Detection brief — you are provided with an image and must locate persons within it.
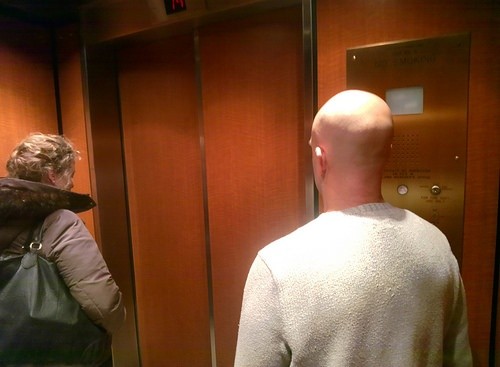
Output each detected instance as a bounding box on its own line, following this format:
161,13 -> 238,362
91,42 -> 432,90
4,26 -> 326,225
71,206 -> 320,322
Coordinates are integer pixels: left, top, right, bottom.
0,133 -> 125,367
234,90 -> 473,367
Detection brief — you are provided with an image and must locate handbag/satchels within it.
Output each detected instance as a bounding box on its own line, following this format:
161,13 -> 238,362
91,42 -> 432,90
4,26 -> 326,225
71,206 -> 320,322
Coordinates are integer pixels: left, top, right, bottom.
0,212 -> 113,367
